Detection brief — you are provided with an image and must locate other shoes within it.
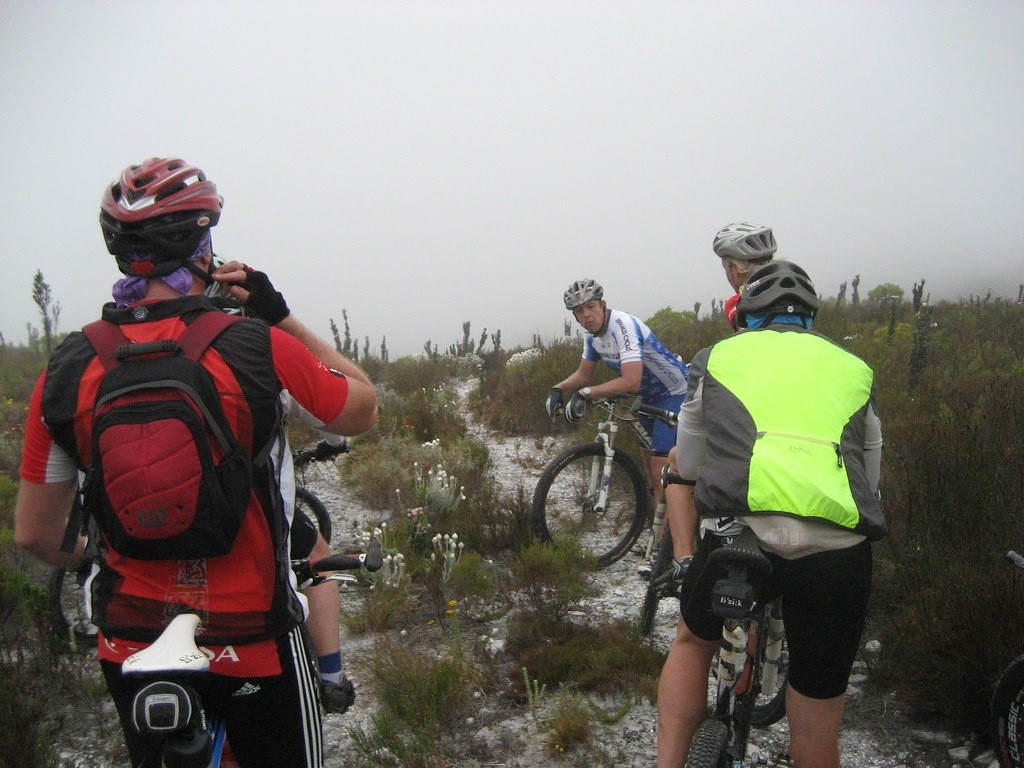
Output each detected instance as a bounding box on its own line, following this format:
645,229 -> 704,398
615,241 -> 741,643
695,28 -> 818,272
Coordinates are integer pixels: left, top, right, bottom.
320,673 -> 356,714
637,565 -> 652,577
652,556 -> 693,593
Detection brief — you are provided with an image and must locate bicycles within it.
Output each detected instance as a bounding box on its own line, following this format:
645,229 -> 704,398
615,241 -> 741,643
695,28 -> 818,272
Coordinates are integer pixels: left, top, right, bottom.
285,440 -> 349,715
532,390 -> 675,569
50,487 -> 332,649
636,402 -> 681,638
686,526 -> 792,768
119,550 -> 382,767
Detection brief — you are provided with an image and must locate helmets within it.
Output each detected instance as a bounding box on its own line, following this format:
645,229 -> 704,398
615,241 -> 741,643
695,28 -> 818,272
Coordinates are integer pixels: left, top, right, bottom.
98,157 -> 224,279
564,278 -> 603,310
737,260 -> 819,328
713,222 -> 778,260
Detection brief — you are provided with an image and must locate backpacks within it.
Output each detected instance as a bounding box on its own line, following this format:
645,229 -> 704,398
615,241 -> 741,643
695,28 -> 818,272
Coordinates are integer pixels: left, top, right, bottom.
59,312 -> 283,561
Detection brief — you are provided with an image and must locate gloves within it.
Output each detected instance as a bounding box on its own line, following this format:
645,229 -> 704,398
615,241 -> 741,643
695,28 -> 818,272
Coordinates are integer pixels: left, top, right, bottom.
545,387 -> 564,422
564,391 -> 590,425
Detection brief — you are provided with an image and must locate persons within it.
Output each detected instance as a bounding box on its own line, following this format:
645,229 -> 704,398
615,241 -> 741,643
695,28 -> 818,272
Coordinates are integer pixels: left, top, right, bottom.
655,220 -> 881,767
17,154 -> 376,768
546,280 -> 689,505
